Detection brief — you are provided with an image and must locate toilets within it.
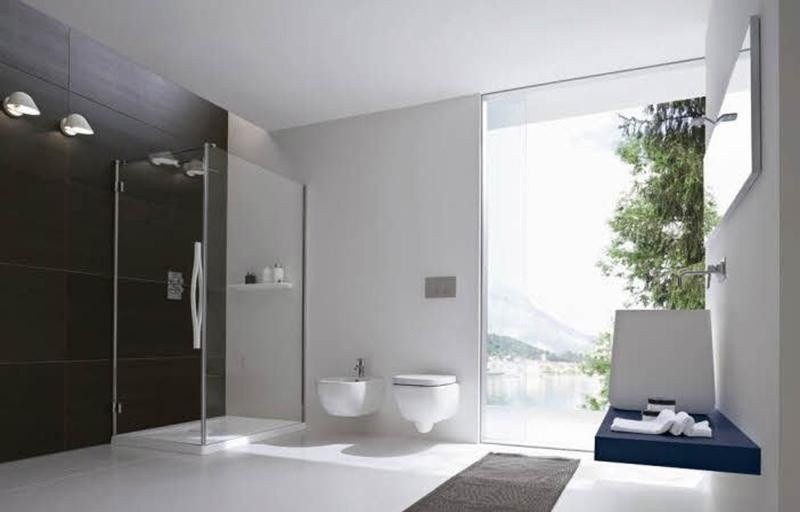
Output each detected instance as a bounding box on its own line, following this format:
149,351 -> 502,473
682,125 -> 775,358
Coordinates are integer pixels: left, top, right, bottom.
392,374 -> 461,433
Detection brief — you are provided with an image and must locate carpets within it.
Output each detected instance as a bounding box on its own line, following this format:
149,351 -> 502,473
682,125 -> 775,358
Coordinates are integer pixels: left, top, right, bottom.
400,448 -> 581,512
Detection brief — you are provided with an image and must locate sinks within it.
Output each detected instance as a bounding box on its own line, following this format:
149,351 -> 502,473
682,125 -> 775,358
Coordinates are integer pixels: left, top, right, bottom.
317,376 -> 385,418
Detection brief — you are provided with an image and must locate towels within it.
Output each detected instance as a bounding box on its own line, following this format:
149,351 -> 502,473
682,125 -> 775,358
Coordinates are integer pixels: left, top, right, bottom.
608,408 -> 713,439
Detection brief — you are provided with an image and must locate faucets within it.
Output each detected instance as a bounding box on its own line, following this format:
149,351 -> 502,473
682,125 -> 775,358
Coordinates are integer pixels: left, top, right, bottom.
351,358 -> 367,377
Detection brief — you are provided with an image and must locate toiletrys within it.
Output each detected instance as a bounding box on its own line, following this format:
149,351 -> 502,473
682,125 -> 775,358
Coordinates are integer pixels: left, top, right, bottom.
274,261 -> 284,283
263,263 -> 273,283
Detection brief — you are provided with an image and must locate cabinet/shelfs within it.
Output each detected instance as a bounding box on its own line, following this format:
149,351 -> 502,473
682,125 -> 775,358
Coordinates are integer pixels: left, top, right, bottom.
3,0 -> 114,462
114,39 -> 228,443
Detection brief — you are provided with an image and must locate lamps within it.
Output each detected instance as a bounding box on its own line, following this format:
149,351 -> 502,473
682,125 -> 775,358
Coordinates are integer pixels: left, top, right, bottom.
58,110 -> 93,136
181,160 -> 205,174
149,149 -> 178,168
4,91 -> 39,119
